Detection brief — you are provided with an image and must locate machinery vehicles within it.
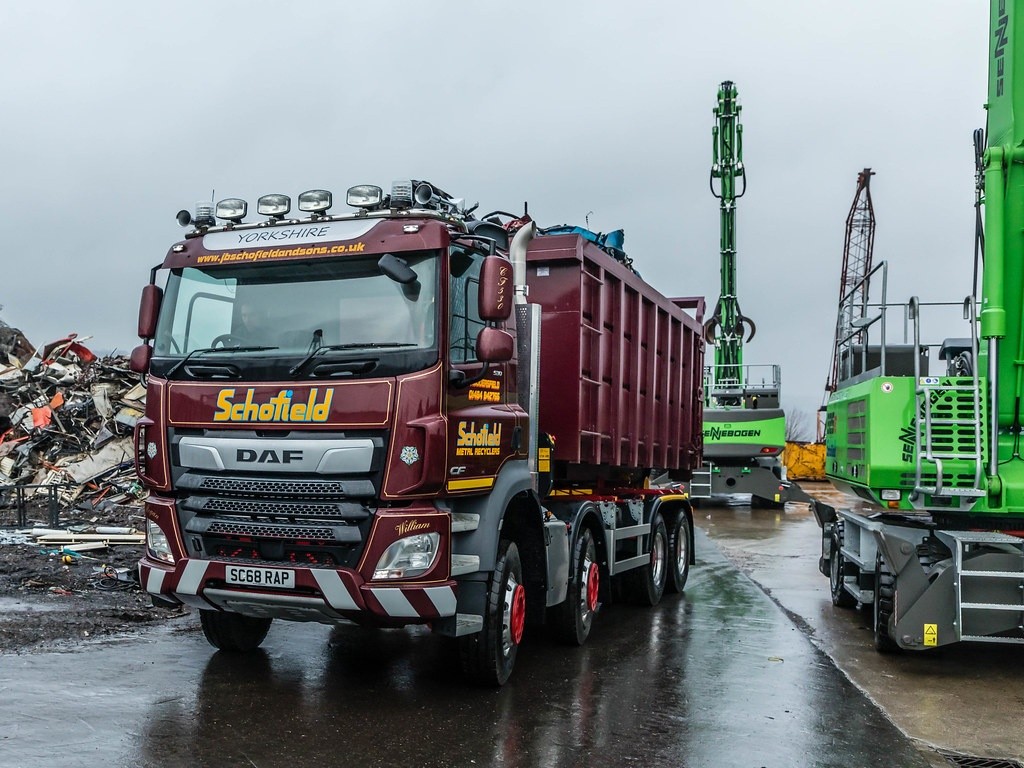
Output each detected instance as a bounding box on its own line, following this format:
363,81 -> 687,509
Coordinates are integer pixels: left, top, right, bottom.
652,81 -> 821,509
808,0 -> 1024,652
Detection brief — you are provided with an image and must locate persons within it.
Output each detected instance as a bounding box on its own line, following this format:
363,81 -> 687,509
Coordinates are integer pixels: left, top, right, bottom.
231,299 -> 275,347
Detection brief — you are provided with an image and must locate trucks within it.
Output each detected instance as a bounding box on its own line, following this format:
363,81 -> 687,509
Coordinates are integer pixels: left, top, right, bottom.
130,178 -> 707,689
